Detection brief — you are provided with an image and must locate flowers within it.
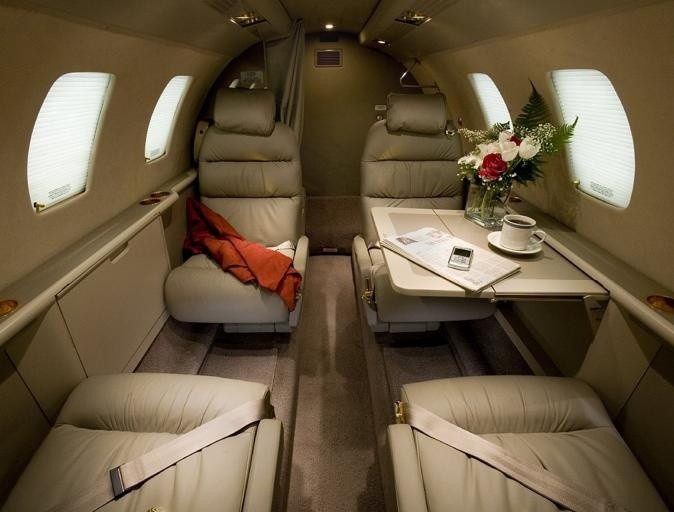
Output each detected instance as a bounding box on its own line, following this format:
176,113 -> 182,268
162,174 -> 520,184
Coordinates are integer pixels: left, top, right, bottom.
457,77 -> 578,221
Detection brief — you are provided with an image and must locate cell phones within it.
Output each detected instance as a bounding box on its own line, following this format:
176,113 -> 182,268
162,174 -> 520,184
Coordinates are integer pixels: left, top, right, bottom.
448,245 -> 474,270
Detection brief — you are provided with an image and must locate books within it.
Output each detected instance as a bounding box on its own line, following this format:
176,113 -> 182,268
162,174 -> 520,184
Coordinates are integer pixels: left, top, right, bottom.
379,226 -> 521,294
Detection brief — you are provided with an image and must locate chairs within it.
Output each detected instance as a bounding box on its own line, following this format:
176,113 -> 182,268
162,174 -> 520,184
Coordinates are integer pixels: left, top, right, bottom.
351,93 -> 496,333
164,88 -> 309,333
386,375 -> 671,512
0,372 -> 284,512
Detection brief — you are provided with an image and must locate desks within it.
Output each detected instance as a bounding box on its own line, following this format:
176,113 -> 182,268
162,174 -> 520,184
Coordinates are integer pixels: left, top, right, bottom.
370,207 -> 609,334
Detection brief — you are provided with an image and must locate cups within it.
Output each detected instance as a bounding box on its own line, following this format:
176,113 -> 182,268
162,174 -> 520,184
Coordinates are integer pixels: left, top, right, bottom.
500,214 -> 546,251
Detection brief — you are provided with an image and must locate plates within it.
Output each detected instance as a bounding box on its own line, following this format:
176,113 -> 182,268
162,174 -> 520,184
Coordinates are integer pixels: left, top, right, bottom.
487,230 -> 542,257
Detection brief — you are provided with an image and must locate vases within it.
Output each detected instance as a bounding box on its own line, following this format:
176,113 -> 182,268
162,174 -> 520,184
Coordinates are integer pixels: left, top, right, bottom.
464,184 -> 513,232
